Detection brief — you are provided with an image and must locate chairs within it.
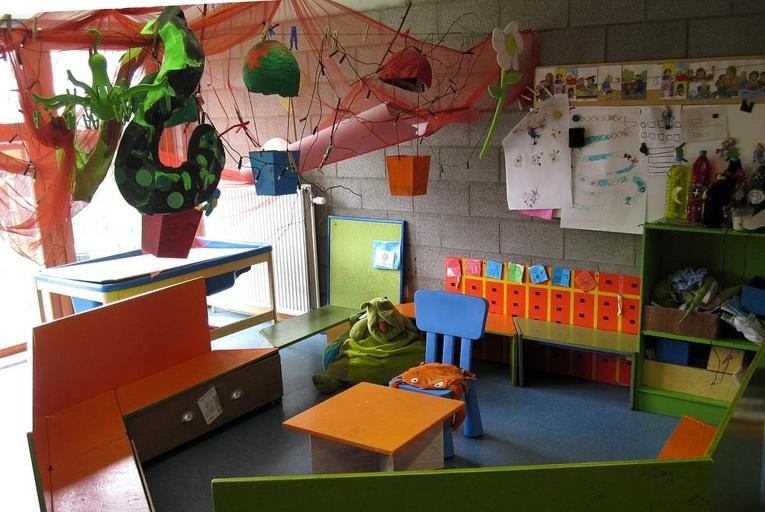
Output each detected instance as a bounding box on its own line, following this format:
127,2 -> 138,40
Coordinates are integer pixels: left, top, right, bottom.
389,289 -> 490,459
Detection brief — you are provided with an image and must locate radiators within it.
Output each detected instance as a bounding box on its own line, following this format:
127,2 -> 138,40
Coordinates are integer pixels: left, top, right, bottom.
202,182 -> 321,317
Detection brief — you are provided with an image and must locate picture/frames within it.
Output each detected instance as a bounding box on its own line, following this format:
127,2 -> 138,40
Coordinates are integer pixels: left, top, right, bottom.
534,56 -> 765,106
326,214 -> 409,310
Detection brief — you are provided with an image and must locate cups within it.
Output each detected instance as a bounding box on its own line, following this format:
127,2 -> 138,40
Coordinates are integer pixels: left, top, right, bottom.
729,209 -> 755,231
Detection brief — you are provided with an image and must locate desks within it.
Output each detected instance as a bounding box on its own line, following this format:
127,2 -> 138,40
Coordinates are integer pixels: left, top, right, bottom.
282,383 -> 467,475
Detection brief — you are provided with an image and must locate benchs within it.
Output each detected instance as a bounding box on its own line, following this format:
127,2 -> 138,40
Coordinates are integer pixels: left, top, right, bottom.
258,304 -> 367,350
655,345 -> 764,512
512,314 -> 639,411
212,456 -> 716,512
392,301 -> 518,390
33,273 -> 283,465
26,330 -> 157,512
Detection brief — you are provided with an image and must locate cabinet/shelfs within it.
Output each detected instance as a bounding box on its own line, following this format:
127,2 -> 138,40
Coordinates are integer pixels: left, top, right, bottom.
636,215 -> 765,424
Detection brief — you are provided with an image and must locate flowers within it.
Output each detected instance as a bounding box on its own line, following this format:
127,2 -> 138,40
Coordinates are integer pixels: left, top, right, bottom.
478,20 -> 527,162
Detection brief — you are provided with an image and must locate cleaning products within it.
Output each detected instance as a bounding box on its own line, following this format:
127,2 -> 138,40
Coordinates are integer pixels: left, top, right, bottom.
664,143 -> 688,220
746,164 -> 764,229
707,160 -> 750,228
687,151 -> 709,226
